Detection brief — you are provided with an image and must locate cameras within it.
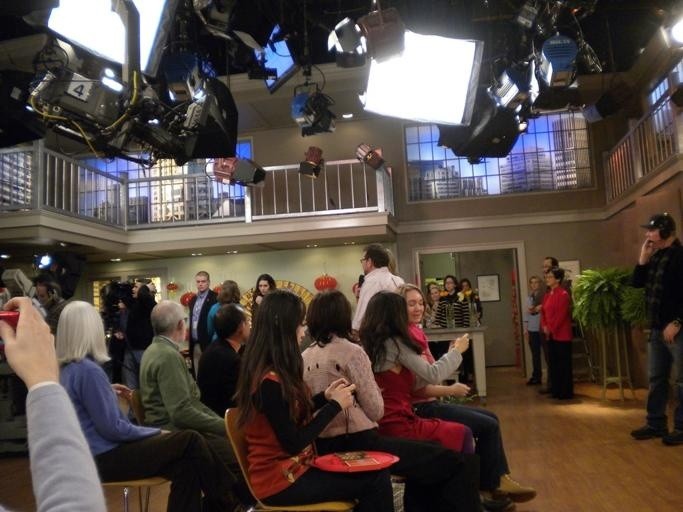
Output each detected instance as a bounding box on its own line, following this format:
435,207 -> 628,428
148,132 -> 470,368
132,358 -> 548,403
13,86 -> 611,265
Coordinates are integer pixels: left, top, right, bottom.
1,312 -> 19,350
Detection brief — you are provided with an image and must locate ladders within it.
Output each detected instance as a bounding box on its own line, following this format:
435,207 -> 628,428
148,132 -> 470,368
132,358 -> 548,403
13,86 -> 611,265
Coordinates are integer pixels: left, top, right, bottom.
564,279 -> 597,384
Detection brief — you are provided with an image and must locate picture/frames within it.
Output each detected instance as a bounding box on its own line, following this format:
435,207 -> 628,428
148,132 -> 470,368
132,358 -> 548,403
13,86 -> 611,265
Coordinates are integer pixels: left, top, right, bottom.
475,272 -> 501,303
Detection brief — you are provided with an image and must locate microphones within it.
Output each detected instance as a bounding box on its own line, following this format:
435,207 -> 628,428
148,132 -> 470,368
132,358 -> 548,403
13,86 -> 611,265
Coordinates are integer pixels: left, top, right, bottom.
647,238 -> 664,244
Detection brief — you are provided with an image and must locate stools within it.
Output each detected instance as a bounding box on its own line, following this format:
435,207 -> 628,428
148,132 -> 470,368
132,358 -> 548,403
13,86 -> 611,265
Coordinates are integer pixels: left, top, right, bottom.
437,369 -> 462,403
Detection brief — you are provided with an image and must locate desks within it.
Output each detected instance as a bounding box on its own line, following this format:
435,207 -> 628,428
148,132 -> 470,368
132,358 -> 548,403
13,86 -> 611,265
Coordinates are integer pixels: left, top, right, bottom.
419,324 -> 489,398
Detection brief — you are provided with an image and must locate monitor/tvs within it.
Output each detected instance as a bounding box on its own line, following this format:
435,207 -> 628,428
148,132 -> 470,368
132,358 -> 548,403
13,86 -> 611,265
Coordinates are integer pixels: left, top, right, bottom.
252,24 -> 300,94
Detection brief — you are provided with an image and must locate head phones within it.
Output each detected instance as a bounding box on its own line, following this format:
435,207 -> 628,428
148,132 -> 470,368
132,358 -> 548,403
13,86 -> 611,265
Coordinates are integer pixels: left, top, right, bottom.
658,210 -> 672,239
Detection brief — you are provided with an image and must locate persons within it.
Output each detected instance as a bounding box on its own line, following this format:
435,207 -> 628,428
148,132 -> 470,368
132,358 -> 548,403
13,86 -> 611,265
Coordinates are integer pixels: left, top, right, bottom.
0,242 -> 536,511
525,255 -> 559,394
539,266 -> 574,400
523,275 -> 546,385
630,212 -> 683,445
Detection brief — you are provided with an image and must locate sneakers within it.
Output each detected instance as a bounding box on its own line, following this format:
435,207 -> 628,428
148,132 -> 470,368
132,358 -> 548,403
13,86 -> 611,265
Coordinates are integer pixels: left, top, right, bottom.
662,431 -> 683,445
481,474 -> 538,512
526,378 -> 574,402
630,421 -> 669,440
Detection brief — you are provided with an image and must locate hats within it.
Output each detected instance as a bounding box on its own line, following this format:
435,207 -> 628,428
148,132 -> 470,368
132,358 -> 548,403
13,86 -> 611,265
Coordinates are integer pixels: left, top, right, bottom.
638,213 -> 676,229
